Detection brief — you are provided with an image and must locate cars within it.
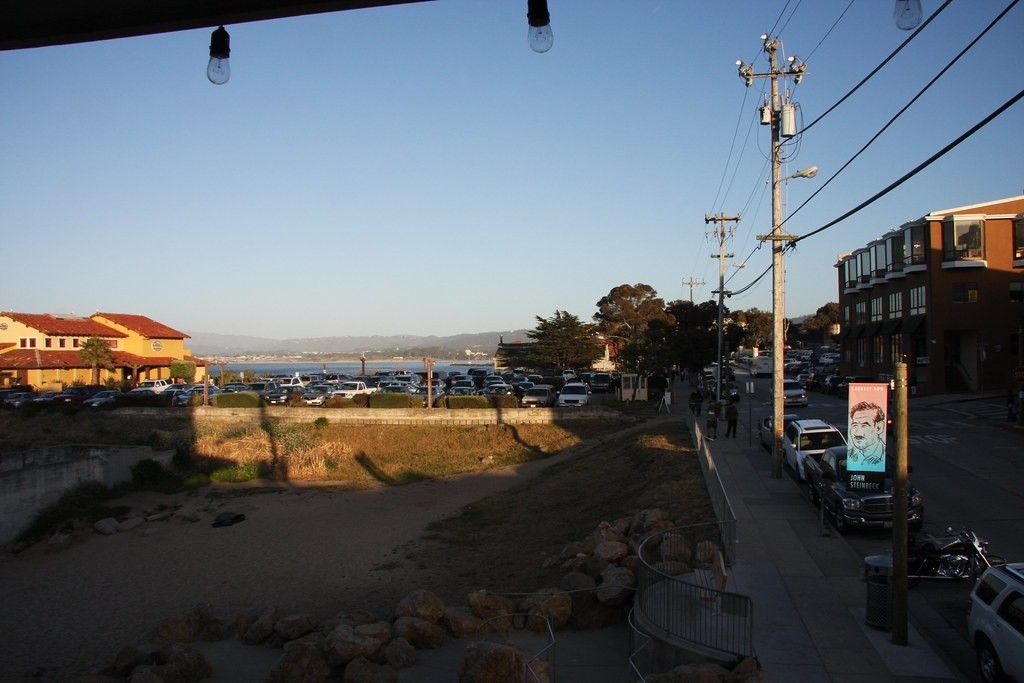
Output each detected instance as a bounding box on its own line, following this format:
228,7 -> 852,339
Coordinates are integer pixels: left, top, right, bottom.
700,343 -> 841,383
714,382 -> 740,403
822,375 -> 844,394
0,368 -> 619,409
795,374 -> 810,386
807,374 -> 824,391
761,415 -> 802,449
837,376 -> 873,398
771,379 -> 808,408
705,380 -> 717,391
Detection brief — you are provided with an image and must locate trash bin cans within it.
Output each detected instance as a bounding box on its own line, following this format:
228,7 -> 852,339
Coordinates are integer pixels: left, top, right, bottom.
863,555 -> 895,632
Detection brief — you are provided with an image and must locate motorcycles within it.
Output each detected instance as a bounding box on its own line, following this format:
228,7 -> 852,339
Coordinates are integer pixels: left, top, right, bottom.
907,526 -> 1006,589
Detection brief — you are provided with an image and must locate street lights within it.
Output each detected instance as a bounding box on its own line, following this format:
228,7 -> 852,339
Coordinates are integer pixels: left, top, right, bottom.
772,166 -> 821,481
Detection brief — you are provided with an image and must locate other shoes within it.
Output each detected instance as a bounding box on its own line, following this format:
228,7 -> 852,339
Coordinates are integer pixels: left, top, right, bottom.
733,436 -> 736,439
1006,418 -> 1018,422
705,437 -> 716,441
724,434 -> 729,439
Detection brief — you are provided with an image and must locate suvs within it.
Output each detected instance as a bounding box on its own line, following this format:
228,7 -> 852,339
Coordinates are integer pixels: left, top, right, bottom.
965,563 -> 1024,683
782,419 -> 847,482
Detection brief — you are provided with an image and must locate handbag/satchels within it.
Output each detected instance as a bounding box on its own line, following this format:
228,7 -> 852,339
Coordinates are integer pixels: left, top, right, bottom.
1012,406 -> 1020,414
1008,403 -> 1012,409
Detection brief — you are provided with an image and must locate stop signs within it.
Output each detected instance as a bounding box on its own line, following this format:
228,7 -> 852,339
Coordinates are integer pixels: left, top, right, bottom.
1012,366 -> 1024,383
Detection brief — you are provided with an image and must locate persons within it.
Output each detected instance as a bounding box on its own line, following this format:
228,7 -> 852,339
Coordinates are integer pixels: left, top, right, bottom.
1005,391 -> 1016,423
724,402 -> 738,439
704,401 -> 720,442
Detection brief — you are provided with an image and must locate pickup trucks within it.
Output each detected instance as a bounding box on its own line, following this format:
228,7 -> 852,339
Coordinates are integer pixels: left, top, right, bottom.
803,446 -> 924,535
